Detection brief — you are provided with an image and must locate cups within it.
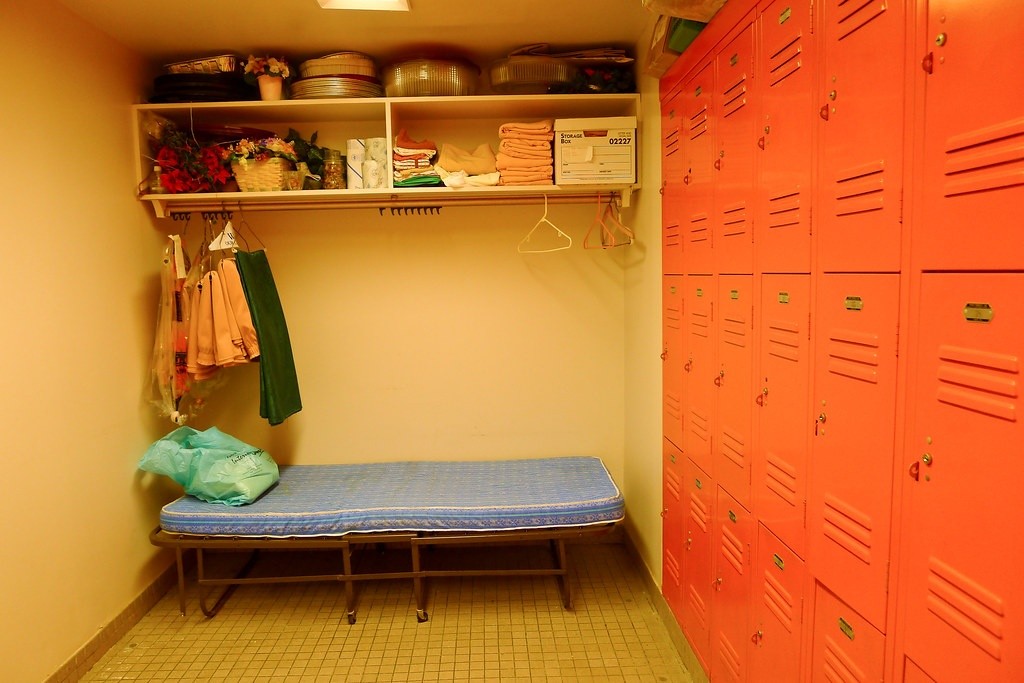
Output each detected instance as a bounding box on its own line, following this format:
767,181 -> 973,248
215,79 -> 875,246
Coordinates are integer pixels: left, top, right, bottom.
283,171 -> 306,191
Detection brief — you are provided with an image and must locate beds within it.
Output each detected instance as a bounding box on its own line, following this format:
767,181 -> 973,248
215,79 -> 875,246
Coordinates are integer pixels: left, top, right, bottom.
149,454 -> 625,624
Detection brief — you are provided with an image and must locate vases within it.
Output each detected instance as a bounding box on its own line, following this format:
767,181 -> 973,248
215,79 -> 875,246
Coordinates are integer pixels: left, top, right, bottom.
256,76 -> 282,100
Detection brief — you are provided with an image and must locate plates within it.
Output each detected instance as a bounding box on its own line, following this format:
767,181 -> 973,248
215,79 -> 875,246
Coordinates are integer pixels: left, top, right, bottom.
290,50 -> 382,99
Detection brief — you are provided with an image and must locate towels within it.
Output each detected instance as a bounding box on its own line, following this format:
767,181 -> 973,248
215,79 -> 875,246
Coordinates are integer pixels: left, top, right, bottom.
392,126 -> 441,188
185,247 -> 304,428
495,119 -> 555,187
433,142 -> 501,189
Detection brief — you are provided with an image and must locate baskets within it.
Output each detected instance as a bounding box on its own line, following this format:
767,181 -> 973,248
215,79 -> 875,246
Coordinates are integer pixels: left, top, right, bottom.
319,52 -> 371,60
230,158 -> 292,192
163,54 -> 236,74
299,58 -> 377,78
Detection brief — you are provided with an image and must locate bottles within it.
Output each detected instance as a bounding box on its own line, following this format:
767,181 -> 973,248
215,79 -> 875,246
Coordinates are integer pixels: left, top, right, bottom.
149,166 -> 166,194
323,150 -> 346,190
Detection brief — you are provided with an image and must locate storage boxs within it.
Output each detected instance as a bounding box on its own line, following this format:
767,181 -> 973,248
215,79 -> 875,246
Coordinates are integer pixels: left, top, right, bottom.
642,0 -> 728,22
667,16 -> 707,53
643,15 -> 679,77
554,116 -> 638,185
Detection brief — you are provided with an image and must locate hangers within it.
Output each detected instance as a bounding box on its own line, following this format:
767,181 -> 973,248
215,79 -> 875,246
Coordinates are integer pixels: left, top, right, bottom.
518,193 -> 572,253
161,200 -> 267,288
583,191 -> 634,249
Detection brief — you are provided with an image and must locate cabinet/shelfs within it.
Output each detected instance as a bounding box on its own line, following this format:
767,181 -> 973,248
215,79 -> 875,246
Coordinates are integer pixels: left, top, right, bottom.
131,92 -> 641,218
661,0 -> 1024,683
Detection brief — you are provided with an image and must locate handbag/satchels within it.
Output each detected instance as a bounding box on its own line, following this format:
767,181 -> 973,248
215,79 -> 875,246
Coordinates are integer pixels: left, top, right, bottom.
138,425 -> 279,507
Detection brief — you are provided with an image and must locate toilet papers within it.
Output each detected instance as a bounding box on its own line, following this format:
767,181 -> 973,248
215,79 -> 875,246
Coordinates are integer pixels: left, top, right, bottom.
346,136 -> 390,189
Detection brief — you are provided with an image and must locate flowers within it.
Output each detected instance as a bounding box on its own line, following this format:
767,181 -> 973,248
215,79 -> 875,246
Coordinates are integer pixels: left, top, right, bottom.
143,116 -> 323,193
239,51 -> 290,81
